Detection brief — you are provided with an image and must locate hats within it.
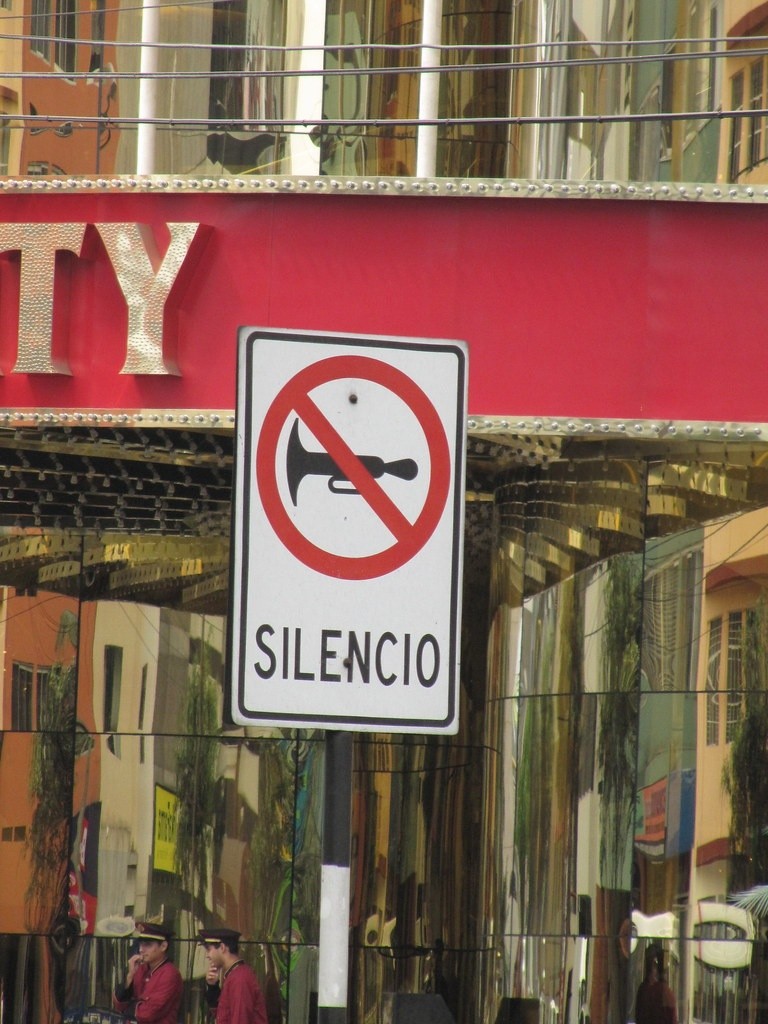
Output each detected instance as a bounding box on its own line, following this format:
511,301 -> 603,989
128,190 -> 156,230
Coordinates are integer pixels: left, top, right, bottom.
198,929 -> 242,946
130,921 -> 175,941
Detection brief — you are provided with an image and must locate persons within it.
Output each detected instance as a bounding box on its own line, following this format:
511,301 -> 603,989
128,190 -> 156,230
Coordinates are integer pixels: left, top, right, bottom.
112,923 -> 185,1024
635,943 -> 676,1024
199,928 -> 268,1024
